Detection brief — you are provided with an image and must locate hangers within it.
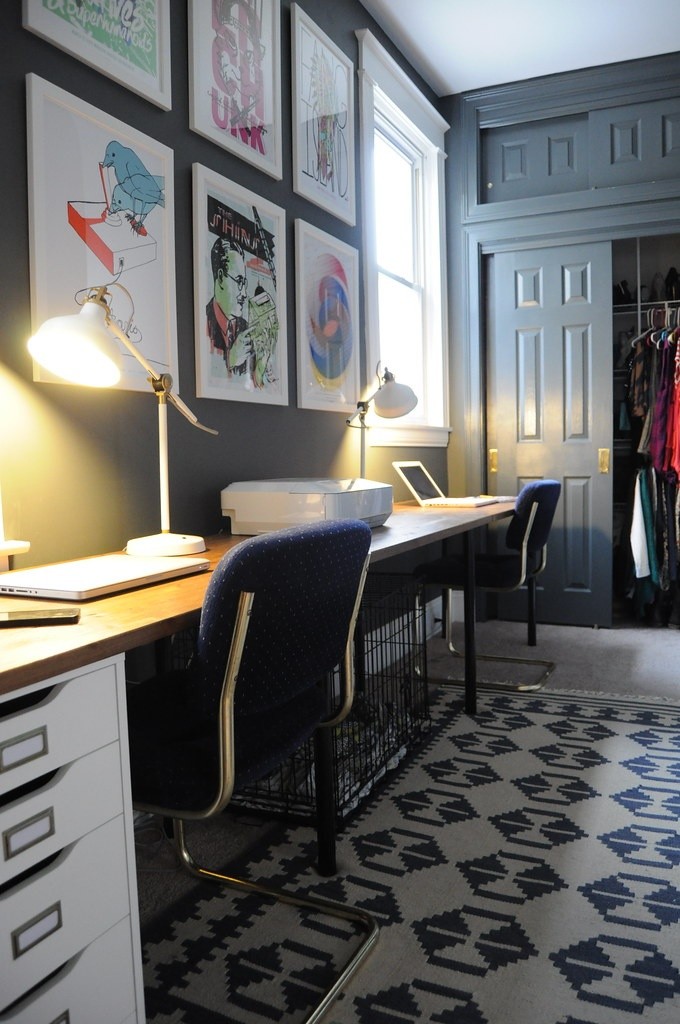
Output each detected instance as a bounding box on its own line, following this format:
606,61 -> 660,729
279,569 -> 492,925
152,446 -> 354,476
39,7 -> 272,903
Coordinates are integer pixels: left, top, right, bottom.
626,305 -> 680,352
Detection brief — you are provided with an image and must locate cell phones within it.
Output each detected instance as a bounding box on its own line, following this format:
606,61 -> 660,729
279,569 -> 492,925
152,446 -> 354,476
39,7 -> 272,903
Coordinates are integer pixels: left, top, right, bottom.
0,608 -> 82,629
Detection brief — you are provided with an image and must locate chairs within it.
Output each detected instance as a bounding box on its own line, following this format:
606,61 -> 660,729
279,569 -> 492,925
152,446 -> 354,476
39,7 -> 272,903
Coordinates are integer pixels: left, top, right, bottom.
418,479 -> 562,694
126,517 -> 382,1024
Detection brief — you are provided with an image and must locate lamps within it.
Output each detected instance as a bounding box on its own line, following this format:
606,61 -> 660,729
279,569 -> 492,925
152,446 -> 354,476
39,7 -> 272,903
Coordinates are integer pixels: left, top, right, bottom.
346,360 -> 419,479
0,283 -> 218,602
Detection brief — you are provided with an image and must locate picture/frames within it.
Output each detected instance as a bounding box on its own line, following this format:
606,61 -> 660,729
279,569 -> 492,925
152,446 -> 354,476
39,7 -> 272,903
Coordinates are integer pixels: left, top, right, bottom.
18,0 -> 363,416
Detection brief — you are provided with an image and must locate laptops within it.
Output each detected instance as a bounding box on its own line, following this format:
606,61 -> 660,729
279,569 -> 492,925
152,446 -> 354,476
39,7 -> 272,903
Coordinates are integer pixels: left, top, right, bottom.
392,461 -> 498,508
0,553 -> 210,599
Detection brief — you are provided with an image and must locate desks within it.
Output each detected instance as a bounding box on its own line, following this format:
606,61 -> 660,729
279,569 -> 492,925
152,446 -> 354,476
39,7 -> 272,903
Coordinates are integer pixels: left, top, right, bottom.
0,494 -> 538,1024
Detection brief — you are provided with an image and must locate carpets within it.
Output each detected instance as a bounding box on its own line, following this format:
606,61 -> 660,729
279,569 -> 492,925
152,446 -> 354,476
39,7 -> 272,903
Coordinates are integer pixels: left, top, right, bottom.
143,675 -> 680,1024
